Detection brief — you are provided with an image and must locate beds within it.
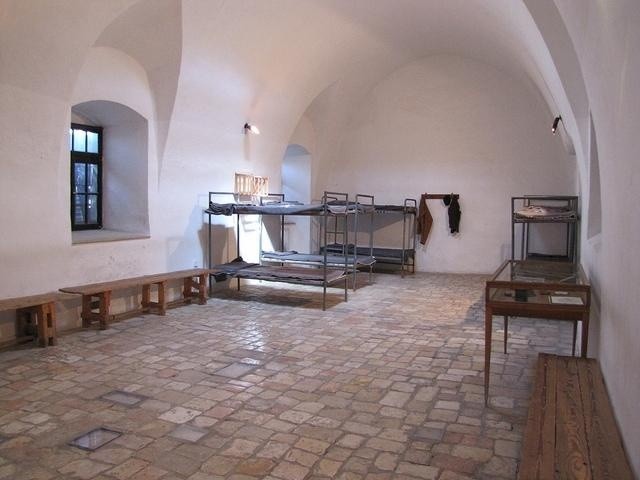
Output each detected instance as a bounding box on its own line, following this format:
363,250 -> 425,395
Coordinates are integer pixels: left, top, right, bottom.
205,187 -> 419,313
506,192 -> 585,285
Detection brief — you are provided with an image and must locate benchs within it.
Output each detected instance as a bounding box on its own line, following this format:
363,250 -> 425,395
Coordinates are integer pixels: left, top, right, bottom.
0,266 -> 218,350
513,350 -> 636,480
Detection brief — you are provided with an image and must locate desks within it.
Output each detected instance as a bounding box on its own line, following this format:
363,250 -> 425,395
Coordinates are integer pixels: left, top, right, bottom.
482,257 -> 595,412
